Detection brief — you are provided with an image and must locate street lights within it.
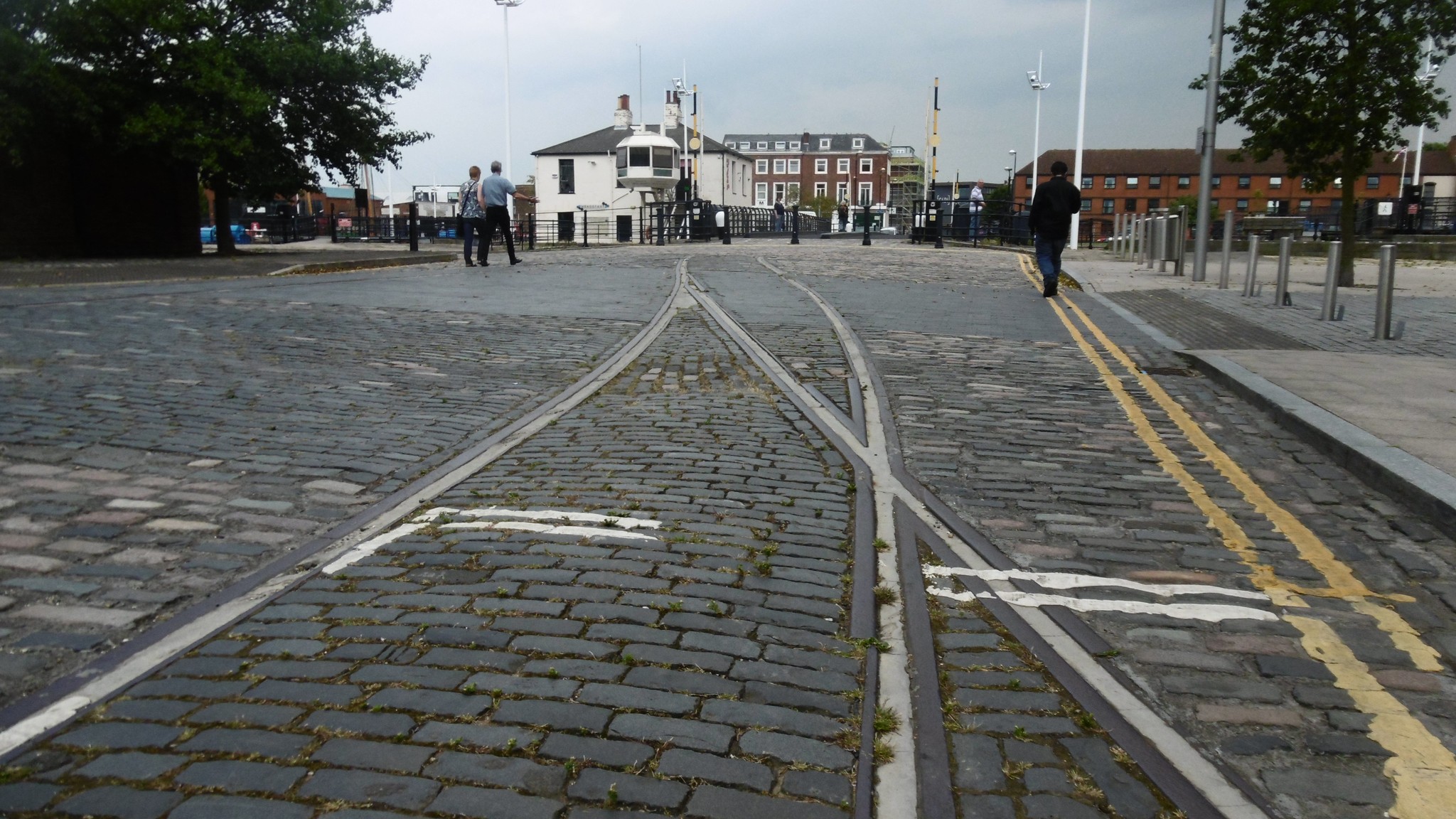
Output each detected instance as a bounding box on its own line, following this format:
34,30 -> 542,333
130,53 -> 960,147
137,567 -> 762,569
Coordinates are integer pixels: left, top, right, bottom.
374,99 -> 401,246
879,167 -> 886,228
853,150 -> 863,231
1004,167 -> 1013,199
672,59 -> 694,240
432,177 -> 441,220
1413,64 -> 1440,187
1009,150 -> 1017,176
495,0 -> 527,247
1026,49 -> 1051,246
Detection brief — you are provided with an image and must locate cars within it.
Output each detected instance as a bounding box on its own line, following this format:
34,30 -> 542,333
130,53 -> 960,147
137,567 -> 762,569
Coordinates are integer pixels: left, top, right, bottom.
1187,219 -> 1225,240
1096,232 -> 1147,243
979,211 -> 1031,238
1304,213 -> 1329,231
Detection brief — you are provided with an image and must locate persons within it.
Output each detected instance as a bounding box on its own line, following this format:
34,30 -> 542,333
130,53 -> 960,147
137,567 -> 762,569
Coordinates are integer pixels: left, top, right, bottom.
1028,161 -> 1081,297
774,197 -> 784,232
458,166 -> 486,267
838,200 -> 846,232
969,180 -> 987,242
481,161 -> 540,265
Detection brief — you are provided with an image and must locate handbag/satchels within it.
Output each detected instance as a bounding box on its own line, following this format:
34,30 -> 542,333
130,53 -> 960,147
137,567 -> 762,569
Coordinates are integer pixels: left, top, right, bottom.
455,214 -> 463,226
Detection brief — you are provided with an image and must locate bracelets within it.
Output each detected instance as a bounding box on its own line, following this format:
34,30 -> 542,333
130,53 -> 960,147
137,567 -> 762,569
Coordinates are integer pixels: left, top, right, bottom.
528,198 -> 530,202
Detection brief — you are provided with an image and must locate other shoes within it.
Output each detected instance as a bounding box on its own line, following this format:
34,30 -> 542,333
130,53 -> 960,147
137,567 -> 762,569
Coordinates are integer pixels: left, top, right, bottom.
1043,282 -> 1058,298
972,239 -> 981,242
839,229 -> 843,232
478,260 -> 490,267
510,258 -> 523,266
465,264 -> 477,267
843,230 -> 847,232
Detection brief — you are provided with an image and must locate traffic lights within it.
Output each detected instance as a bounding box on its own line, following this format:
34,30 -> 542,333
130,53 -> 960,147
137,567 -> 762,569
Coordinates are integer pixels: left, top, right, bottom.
682,177 -> 690,191
